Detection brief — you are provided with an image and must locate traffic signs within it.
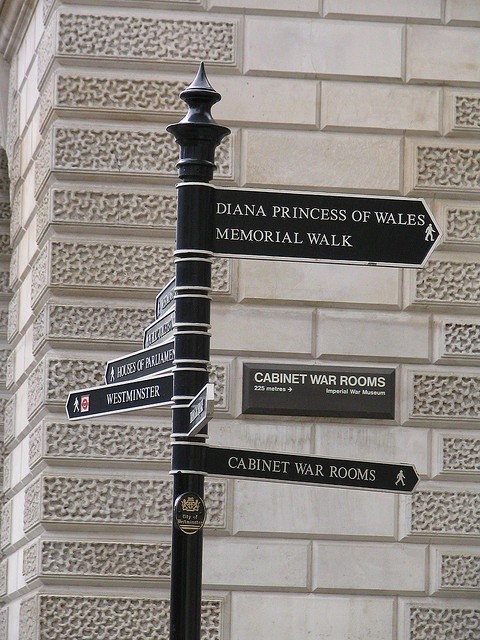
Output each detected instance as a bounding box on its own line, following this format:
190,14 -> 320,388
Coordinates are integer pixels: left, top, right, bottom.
155,276 -> 177,321
143,308 -> 176,349
103,339 -> 174,385
215,186 -> 443,269
187,383 -> 214,435
64,372 -> 174,422
207,444 -> 420,494
242,362 -> 395,420
